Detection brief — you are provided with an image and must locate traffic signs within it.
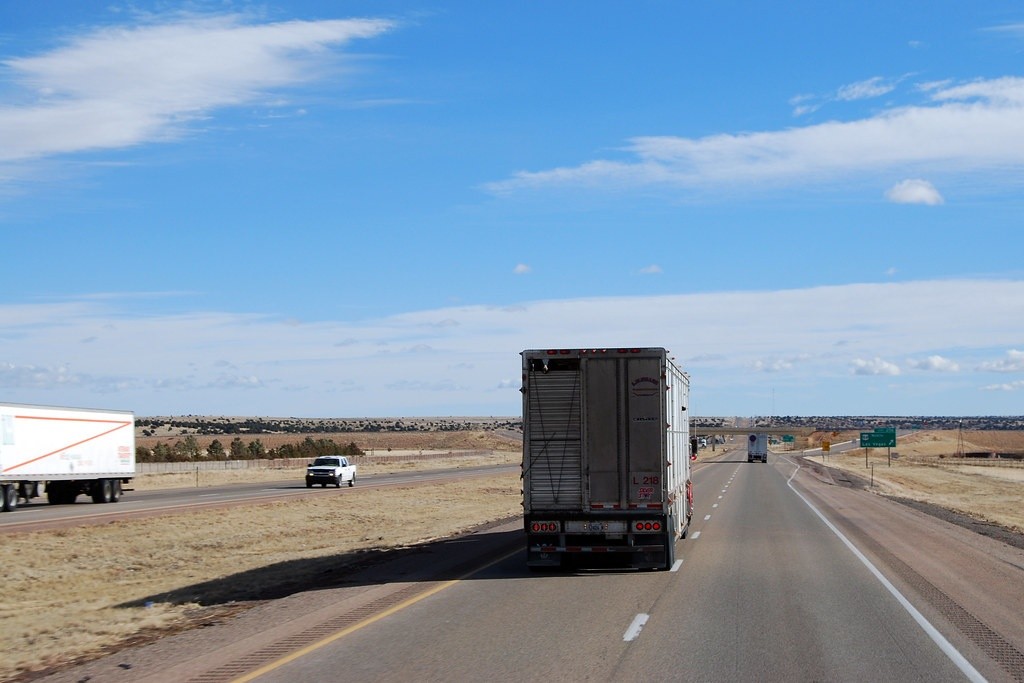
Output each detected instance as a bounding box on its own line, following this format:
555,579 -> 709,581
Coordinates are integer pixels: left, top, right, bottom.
859,426 -> 897,448
781,434 -> 794,443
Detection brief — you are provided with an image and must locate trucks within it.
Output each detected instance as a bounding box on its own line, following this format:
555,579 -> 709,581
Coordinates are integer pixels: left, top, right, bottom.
747,430 -> 769,463
518,346 -> 699,573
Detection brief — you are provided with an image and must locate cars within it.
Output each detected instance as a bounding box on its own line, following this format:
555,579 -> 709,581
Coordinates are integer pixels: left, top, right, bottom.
697,438 -> 706,448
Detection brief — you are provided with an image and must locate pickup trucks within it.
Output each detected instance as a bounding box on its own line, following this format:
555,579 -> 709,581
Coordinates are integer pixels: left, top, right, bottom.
306,454 -> 360,492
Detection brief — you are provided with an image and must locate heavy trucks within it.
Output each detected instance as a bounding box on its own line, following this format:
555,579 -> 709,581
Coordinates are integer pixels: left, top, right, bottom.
0,401 -> 137,512
